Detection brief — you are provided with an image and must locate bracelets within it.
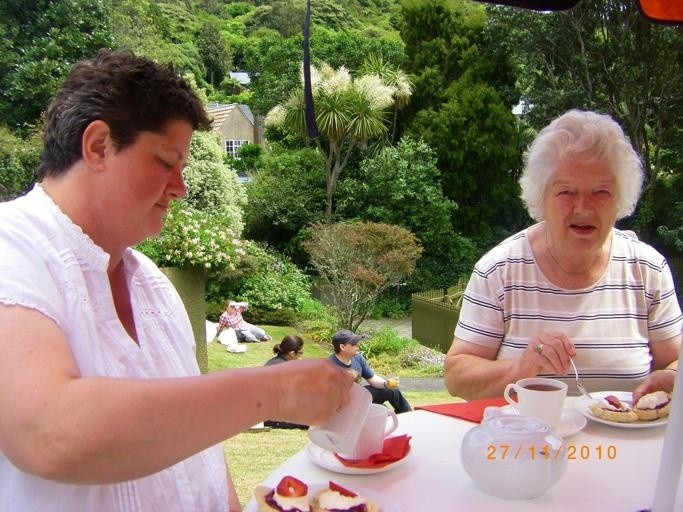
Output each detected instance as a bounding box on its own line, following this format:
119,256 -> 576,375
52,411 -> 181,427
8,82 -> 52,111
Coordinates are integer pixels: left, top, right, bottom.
665,368 -> 677,371
384,382 -> 390,391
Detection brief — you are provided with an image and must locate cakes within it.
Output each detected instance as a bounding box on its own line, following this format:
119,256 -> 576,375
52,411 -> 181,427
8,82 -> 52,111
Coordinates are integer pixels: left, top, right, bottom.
589,396 -> 639,422
254,475 -> 311,512
310,481 -> 379,512
633,391 -> 671,420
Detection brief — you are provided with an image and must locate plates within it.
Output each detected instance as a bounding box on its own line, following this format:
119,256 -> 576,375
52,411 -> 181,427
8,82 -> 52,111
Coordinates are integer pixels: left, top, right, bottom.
490,406 -> 587,439
312,438 -> 420,474
571,392 -> 672,428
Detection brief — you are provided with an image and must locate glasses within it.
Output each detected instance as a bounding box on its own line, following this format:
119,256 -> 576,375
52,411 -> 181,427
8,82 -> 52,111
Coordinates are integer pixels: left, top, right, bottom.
296,351 -> 303,354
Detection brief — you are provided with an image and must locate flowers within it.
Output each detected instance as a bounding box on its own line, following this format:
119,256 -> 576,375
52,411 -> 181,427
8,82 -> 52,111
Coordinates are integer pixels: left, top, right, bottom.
131,199 -> 248,271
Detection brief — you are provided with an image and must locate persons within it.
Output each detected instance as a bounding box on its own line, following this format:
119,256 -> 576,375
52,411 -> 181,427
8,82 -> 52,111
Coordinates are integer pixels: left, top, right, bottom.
327,329 -> 413,414
0,47 -> 355,512
443,107 -> 683,406
215,300 -> 272,343
262,335 -> 311,430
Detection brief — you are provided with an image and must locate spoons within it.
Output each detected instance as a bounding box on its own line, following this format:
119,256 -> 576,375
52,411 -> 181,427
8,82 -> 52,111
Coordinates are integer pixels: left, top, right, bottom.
564,352 -> 595,404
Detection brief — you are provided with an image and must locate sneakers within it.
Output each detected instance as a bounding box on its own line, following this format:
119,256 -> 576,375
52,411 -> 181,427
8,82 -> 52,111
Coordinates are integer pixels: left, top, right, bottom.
264,335 -> 272,341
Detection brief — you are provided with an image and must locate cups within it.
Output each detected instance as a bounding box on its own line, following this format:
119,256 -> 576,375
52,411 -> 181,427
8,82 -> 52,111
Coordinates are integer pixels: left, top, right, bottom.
502,377 -> 569,424
308,376 -> 397,461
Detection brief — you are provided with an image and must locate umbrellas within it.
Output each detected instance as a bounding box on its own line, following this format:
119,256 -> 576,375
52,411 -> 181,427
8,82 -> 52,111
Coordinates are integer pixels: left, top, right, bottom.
474,0 -> 683,26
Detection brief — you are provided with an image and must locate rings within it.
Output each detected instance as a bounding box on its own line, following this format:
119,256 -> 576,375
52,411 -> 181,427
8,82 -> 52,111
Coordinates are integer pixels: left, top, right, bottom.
336,403 -> 343,412
537,344 -> 543,353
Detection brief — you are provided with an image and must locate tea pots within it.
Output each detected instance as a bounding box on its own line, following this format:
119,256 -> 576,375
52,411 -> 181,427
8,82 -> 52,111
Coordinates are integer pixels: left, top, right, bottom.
456,412 -> 567,499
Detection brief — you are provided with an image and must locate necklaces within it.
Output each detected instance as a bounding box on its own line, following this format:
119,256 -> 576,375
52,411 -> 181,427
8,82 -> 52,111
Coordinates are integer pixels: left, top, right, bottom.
544,230 -> 598,276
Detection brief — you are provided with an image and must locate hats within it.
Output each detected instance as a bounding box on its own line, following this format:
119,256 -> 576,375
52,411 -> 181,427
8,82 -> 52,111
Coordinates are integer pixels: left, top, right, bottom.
331,329 -> 365,345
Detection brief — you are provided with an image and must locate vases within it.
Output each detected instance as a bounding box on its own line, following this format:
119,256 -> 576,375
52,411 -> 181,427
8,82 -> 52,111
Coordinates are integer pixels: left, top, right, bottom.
158,265 -> 209,373
